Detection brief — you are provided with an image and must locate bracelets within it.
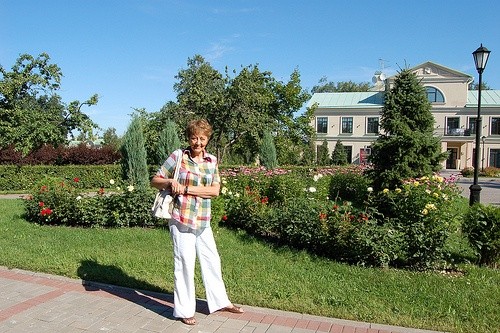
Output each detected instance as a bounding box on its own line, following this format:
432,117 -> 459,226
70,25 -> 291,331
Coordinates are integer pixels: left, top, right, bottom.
183,184 -> 190,195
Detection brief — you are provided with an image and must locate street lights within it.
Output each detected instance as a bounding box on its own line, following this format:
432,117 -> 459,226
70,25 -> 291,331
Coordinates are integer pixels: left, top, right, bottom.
469,42 -> 492,207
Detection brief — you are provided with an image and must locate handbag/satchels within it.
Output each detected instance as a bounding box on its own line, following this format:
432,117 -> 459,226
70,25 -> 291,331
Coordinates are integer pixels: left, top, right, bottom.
152,148 -> 182,219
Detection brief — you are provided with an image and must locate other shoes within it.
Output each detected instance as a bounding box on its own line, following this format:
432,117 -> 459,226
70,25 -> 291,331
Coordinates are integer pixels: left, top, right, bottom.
219,305 -> 244,312
182,318 -> 196,325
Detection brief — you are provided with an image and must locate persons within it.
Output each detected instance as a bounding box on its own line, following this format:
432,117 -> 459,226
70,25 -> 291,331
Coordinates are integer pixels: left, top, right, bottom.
149,117 -> 245,324
